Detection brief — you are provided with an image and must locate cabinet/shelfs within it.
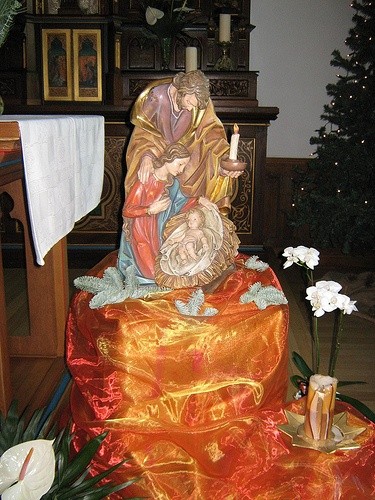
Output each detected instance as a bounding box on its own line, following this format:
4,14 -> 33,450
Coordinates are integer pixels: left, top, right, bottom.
1,0 -> 279,259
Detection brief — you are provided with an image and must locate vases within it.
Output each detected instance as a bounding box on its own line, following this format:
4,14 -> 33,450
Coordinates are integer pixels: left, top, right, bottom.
159,39 -> 173,71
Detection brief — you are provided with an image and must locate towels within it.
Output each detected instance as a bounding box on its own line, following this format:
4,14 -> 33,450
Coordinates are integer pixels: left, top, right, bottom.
0,114 -> 107,267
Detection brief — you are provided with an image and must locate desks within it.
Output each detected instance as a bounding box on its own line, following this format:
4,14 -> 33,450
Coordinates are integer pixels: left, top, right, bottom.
0,122 -> 71,424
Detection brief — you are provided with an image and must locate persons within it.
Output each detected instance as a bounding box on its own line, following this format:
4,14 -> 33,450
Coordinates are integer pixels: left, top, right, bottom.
116,70 -> 244,285
167,208 -> 209,265
121,143 -> 220,280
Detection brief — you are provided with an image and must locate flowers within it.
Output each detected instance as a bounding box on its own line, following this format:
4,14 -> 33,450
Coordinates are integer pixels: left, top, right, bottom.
283,245 -> 358,400
133,0 -> 205,38
1,367 -> 141,499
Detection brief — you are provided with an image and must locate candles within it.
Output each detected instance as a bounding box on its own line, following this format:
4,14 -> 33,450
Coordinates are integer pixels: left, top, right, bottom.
305,373 -> 338,441
218,14 -> 231,42
185,47 -> 197,73
